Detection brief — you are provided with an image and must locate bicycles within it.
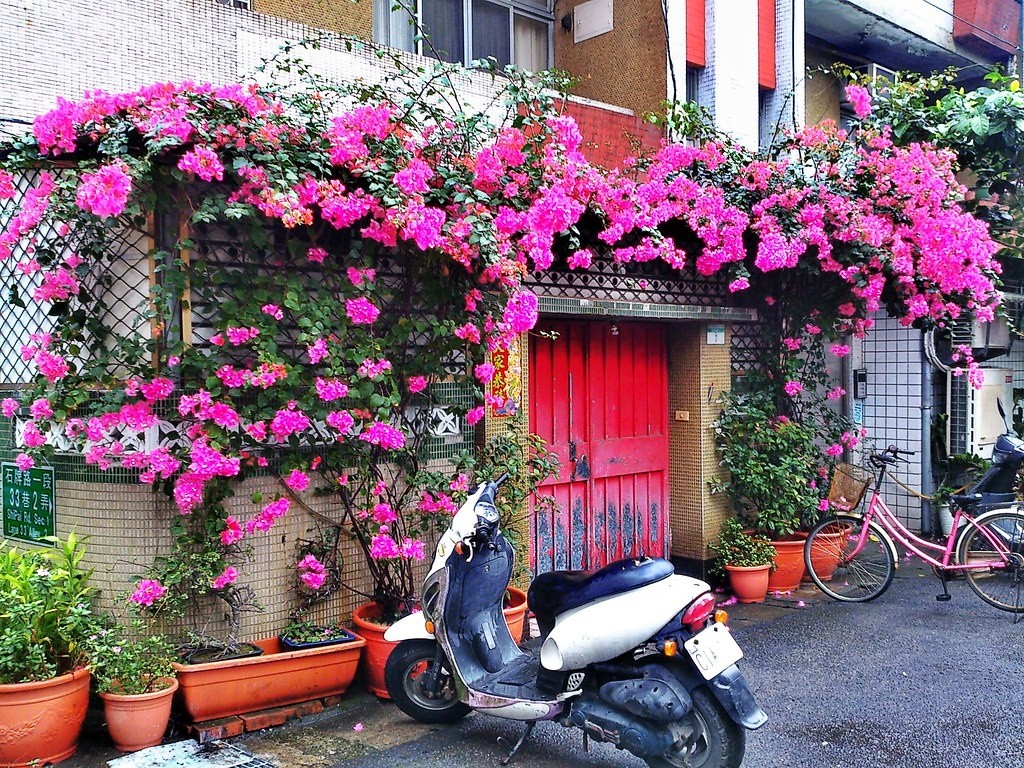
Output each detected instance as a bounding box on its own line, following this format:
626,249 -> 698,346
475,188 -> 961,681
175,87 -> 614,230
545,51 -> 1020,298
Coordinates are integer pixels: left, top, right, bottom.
803,444 -> 1024,624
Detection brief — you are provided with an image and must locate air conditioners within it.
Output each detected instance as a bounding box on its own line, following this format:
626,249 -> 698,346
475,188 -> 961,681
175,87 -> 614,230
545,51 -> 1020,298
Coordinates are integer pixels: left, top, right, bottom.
945,368 -> 1014,460
839,63 -> 897,110
950,296 -> 1010,351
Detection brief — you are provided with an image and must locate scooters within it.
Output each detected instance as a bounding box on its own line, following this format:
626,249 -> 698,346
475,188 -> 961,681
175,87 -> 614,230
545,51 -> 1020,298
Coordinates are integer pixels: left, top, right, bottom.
383,470 -> 769,768
952,395 -> 1024,557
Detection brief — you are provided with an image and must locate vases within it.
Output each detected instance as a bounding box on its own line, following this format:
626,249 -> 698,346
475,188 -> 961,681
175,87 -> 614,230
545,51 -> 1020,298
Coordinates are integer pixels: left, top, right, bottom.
969,185 -> 993,201
98,675 -> 179,752
796,520 -> 852,583
165,601 -> 428,722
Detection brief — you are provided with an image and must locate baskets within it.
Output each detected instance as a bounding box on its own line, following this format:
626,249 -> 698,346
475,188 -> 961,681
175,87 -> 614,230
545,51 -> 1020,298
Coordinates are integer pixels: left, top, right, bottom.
827,461 -> 875,511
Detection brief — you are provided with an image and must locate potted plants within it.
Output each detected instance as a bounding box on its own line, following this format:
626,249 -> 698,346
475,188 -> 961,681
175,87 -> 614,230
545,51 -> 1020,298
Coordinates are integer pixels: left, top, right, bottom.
0,531 -> 104,768
936,487 -> 967,537
706,389 -> 823,604
411,407 -> 564,646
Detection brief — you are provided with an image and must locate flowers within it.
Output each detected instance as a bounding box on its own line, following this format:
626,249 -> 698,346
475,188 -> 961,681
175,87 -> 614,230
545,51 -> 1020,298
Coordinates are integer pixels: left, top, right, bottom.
78,601 -> 180,694
